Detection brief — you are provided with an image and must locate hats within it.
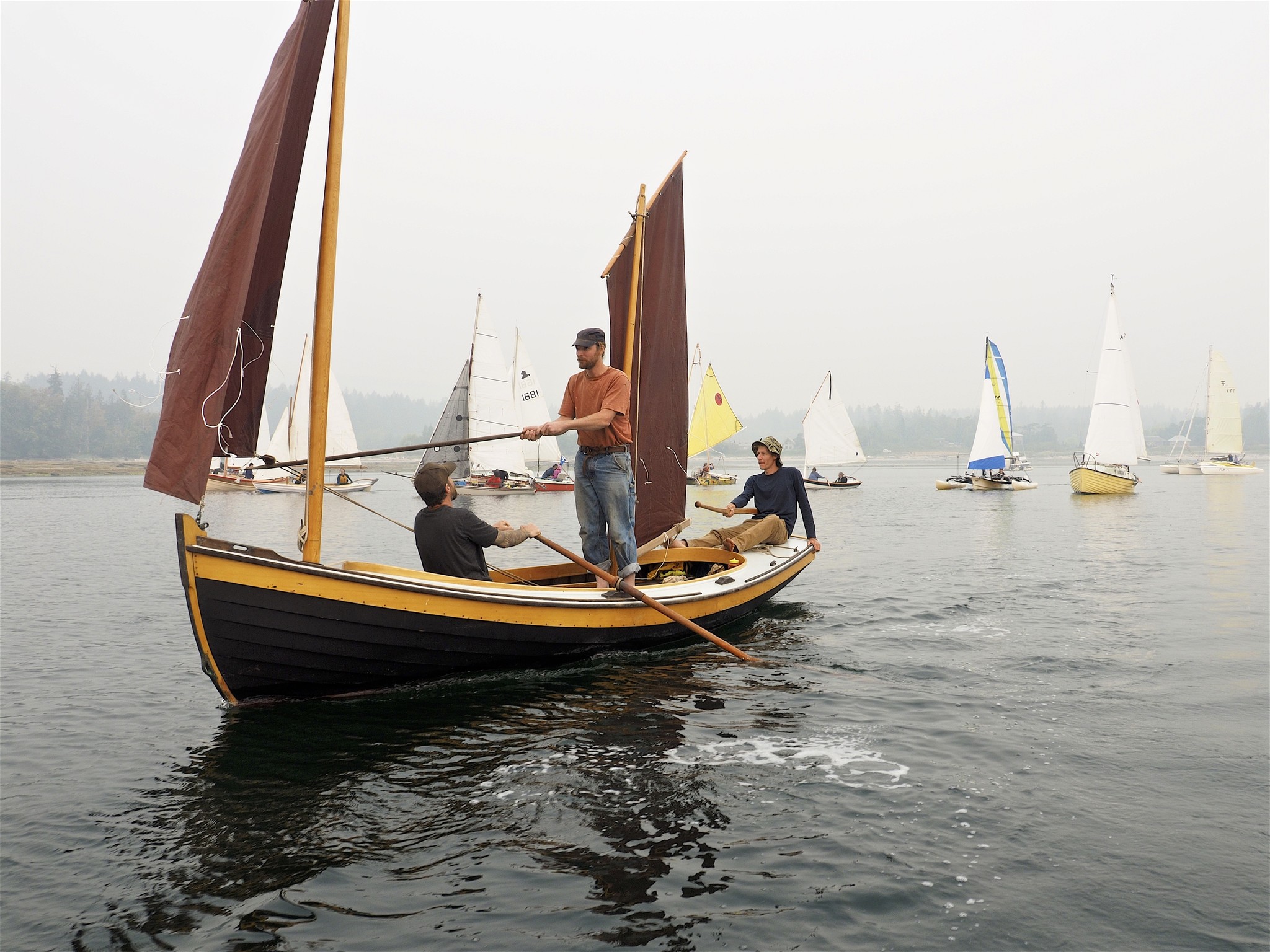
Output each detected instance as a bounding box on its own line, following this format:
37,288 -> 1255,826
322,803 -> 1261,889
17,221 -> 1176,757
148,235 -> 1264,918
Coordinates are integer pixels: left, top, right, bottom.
751,437 -> 783,466
414,461 -> 457,498
553,464 -> 558,467
571,328 -> 606,347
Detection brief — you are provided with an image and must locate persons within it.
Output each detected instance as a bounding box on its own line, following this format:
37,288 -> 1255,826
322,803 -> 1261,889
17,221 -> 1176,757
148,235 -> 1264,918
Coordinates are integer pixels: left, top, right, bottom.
242,463 -> 254,480
833,472 -> 847,483
541,464 -> 562,480
334,468 -> 353,486
992,468 -> 1006,480
483,469 -> 509,488
521,327 -> 641,590
413,461 -> 541,582
1233,455 -> 1239,464
294,468 -> 307,485
1225,454 -> 1233,462
701,463 -> 714,477
808,467 -> 827,481
661,436 -> 821,554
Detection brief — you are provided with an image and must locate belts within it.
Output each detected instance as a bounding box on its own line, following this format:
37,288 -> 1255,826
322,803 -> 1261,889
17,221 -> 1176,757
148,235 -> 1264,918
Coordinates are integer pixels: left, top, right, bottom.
578,444 -> 630,475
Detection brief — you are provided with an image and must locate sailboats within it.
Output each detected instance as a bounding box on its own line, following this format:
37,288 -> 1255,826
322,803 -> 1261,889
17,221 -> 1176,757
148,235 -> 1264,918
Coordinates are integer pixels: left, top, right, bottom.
935,335 -> 1039,491
1159,343 -> 1264,475
801,368 -> 869,490
1068,274 -> 1152,496
687,342 -> 747,487
204,334 -> 379,495
142,0 -> 816,708
380,288 -> 574,495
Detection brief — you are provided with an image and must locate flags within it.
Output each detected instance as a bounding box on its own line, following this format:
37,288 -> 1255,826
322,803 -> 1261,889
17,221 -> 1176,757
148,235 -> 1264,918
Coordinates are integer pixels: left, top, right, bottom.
559,455 -> 566,466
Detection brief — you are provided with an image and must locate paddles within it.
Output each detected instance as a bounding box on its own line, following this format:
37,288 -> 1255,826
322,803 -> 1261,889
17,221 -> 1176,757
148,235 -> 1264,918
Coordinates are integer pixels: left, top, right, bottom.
826,478 -> 831,489
534,534 -> 755,661
